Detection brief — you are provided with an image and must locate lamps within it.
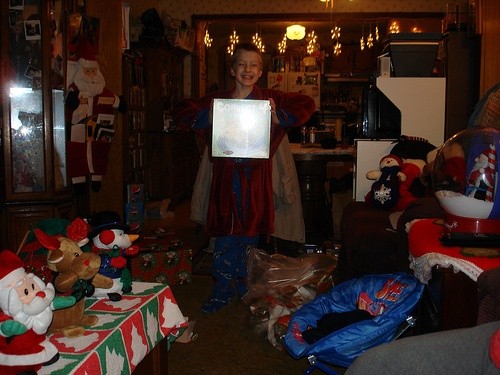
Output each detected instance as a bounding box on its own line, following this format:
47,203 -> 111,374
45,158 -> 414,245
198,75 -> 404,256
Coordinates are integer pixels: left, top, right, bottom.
285,24 -> 306,40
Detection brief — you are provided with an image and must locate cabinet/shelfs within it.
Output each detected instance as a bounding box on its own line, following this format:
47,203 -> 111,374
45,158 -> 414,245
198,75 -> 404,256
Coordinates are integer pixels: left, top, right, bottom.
268,33 -> 482,257
124,40 -> 196,211
0,0 -> 87,252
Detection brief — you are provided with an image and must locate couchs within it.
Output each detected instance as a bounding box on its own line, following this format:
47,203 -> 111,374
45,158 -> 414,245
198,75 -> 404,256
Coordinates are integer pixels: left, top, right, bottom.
340,140 -> 441,281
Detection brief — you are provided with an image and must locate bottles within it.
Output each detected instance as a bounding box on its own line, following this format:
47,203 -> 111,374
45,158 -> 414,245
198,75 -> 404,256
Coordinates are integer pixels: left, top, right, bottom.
301,126 -> 316,144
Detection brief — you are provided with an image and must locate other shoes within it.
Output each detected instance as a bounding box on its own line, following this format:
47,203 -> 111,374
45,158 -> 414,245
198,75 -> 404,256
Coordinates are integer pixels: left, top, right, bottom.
234,280 -> 248,296
199,295 -> 232,313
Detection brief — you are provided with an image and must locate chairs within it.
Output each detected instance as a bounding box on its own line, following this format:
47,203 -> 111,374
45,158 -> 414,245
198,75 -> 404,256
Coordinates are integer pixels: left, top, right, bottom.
282,273 -> 425,375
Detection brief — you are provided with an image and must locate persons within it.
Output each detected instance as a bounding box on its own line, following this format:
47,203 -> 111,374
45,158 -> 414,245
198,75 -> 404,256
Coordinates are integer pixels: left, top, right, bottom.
174,43 -> 316,314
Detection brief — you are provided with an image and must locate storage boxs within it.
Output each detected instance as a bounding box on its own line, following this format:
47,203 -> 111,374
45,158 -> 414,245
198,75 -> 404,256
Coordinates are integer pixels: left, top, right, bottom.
126,230 -> 192,297
381,42 -> 439,77
380,57 -> 390,77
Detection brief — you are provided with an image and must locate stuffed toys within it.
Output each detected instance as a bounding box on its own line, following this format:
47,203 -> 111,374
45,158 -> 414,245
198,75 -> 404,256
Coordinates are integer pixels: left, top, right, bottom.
60,57 -> 127,194
366,154 -> 406,209
0,211 -> 139,375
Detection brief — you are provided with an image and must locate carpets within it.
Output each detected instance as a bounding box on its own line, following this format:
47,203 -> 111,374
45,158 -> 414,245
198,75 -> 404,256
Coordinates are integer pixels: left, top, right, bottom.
188,238 -> 214,275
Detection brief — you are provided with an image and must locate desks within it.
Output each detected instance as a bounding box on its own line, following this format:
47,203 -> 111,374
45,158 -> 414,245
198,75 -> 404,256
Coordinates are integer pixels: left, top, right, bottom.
405,219 -> 500,330
36,281 -> 189,375
